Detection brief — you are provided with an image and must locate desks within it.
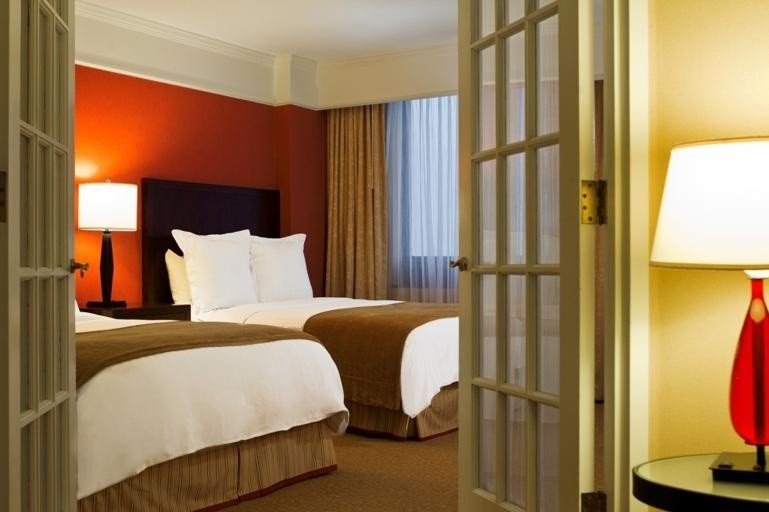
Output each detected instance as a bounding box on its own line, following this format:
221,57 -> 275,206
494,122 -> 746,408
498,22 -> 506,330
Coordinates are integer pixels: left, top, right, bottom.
77,178 -> 138,308
632,453 -> 769,512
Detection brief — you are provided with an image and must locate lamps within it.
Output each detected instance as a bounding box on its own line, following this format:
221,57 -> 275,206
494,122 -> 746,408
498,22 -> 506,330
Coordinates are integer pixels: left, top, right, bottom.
649,135 -> 769,485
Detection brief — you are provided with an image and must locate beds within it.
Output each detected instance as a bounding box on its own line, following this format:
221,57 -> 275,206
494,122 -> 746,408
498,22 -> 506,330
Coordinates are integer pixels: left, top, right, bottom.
141,176 -> 459,442
73,300 -> 350,512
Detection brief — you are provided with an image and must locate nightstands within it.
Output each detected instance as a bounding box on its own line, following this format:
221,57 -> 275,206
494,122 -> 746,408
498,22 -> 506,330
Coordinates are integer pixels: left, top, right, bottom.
79,304 -> 191,322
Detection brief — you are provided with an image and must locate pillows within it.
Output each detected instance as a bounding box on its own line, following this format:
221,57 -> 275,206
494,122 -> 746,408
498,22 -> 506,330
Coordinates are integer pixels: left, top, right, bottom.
164,249 -> 192,306
170,228 -> 257,314
250,233 -> 314,303
